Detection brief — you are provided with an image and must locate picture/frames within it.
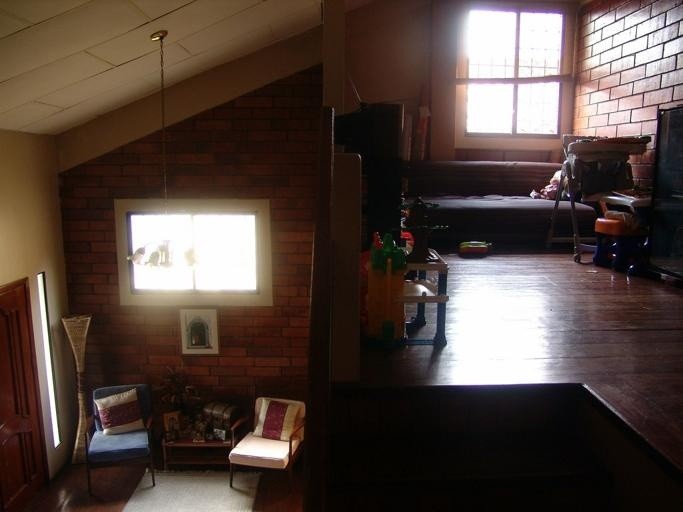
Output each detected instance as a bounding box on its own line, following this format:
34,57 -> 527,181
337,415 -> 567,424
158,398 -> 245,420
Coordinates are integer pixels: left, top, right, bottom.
179,309 -> 218,355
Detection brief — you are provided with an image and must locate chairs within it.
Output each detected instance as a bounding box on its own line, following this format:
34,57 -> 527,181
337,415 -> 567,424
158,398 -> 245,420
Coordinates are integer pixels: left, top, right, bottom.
228,396 -> 306,495
84,383 -> 155,494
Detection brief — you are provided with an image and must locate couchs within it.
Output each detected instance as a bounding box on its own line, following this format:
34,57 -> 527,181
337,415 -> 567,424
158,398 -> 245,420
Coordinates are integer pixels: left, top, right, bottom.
147,393 -> 256,470
405,159 -> 596,248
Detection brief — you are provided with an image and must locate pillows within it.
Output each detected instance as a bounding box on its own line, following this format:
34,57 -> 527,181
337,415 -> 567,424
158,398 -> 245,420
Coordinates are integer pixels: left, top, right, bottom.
93,388 -> 146,436
253,398 -> 300,441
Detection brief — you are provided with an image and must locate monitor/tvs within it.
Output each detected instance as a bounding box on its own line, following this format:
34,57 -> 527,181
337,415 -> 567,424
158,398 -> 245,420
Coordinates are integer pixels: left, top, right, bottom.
335,101 -> 405,172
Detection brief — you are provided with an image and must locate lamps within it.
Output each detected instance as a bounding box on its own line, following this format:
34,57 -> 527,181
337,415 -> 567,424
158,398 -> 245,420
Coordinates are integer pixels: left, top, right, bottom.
126,30 -> 198,269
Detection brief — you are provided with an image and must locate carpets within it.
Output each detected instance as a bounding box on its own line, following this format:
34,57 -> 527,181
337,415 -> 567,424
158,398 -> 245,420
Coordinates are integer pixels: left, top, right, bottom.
301,382 -> 683,512
121,467 -> 262,512
